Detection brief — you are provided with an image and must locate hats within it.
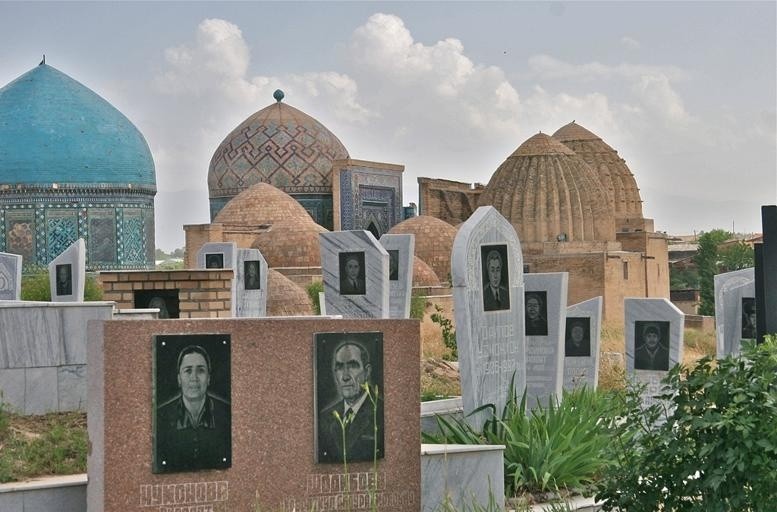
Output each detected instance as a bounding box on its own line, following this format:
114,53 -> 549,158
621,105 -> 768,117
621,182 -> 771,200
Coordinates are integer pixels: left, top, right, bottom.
643,326 -> 660,336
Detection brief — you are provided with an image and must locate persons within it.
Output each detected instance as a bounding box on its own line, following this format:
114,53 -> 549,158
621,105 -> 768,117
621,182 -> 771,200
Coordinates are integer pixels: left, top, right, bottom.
742,308 -> 756,337
387,253 -> 397,279
147,296 -> 170,318
565,321 -> 589,356
155,343 -> 230,470
246,263 -> 259,289
340,256 -> 364,294
525,293 -> 546,334
207,256 -> 220,268
481,249 -> 508,310
316,341 -> 384,463
635,324 -> 668,370
56,266 -> 71,294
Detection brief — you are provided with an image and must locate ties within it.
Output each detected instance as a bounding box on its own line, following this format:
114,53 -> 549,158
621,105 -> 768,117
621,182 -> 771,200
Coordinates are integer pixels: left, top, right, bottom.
343,408 -> 354,432
495,291 -> 499,303
353,280 -> 357,289
